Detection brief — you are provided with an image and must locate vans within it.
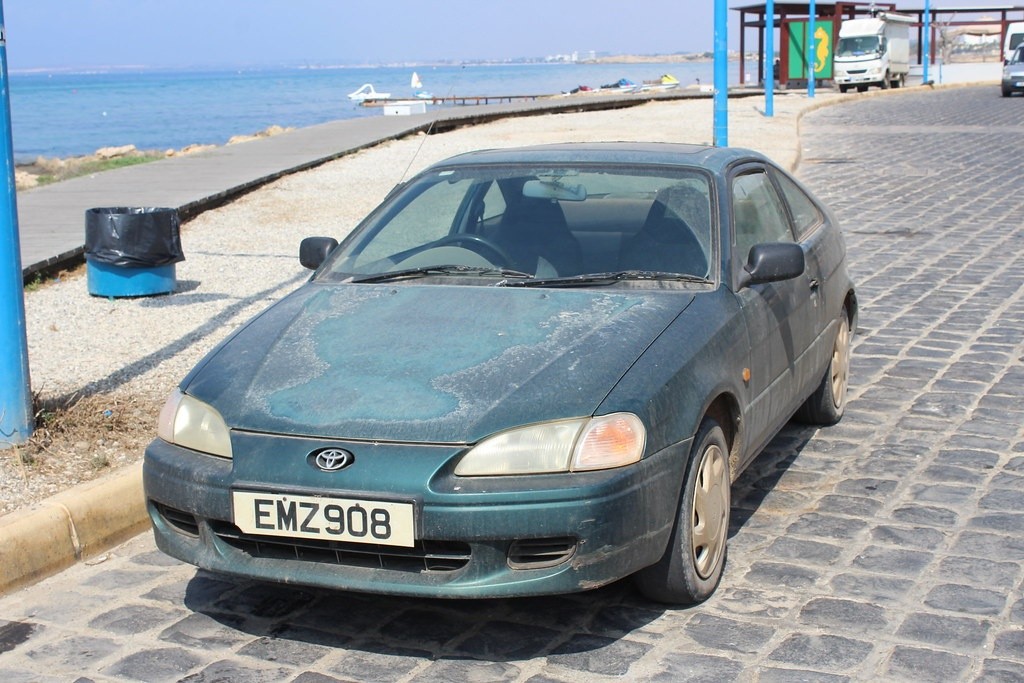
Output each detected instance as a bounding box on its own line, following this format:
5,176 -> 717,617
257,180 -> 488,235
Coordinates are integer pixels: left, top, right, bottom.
1003,22 -> 1024,63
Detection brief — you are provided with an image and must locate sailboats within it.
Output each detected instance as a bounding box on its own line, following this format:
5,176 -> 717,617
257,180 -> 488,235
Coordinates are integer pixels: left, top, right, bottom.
411,73 -> 435,99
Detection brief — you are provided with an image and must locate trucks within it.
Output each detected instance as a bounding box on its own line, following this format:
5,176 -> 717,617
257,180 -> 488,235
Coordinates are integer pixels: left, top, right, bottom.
833,12 -> 915,93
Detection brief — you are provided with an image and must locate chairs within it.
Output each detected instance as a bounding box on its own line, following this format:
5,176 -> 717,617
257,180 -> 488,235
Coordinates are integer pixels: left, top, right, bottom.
486,196 -> 583,276
622,188 -> 709,277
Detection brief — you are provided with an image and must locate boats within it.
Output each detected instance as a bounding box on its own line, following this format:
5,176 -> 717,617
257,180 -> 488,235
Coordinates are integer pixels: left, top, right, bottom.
561,85 -> 596,93
599,79 -> 636,93
642,74 -> 679,91
348,84 -> 392,101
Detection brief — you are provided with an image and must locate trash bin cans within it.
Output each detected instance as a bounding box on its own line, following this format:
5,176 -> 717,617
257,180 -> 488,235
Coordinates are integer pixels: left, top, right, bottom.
85,206 -> 185,302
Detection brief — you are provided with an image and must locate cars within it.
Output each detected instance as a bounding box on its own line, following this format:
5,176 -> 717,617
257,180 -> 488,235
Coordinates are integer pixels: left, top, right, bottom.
143,140 -> 858,607
1002,41 -> 1024,97
